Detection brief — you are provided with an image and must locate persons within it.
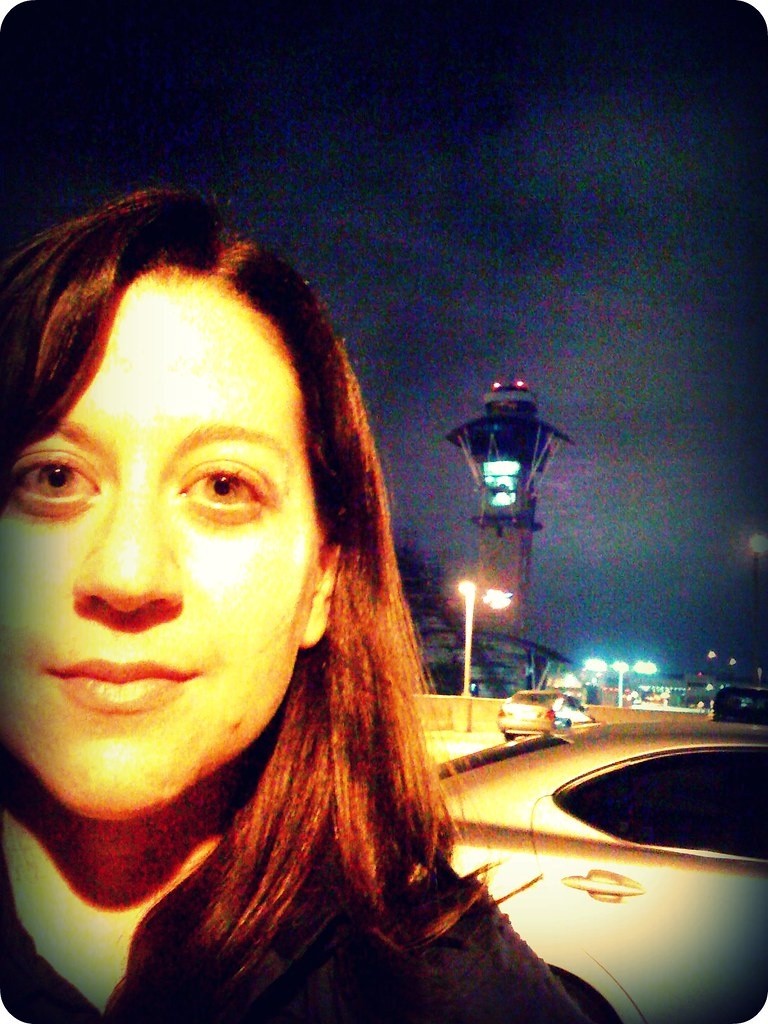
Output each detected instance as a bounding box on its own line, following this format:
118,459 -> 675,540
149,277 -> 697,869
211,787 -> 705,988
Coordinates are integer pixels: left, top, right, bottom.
0,186 -> 592,1024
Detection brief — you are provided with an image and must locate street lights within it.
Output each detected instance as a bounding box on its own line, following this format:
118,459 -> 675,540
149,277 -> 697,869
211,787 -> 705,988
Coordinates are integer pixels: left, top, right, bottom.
458,580 -> 477,698
614,661 -> 629,709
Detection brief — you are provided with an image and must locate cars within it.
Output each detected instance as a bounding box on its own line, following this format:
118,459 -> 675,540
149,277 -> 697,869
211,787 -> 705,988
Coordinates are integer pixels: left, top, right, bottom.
401,722 -> 768,1024
495,690 -> 597,743
